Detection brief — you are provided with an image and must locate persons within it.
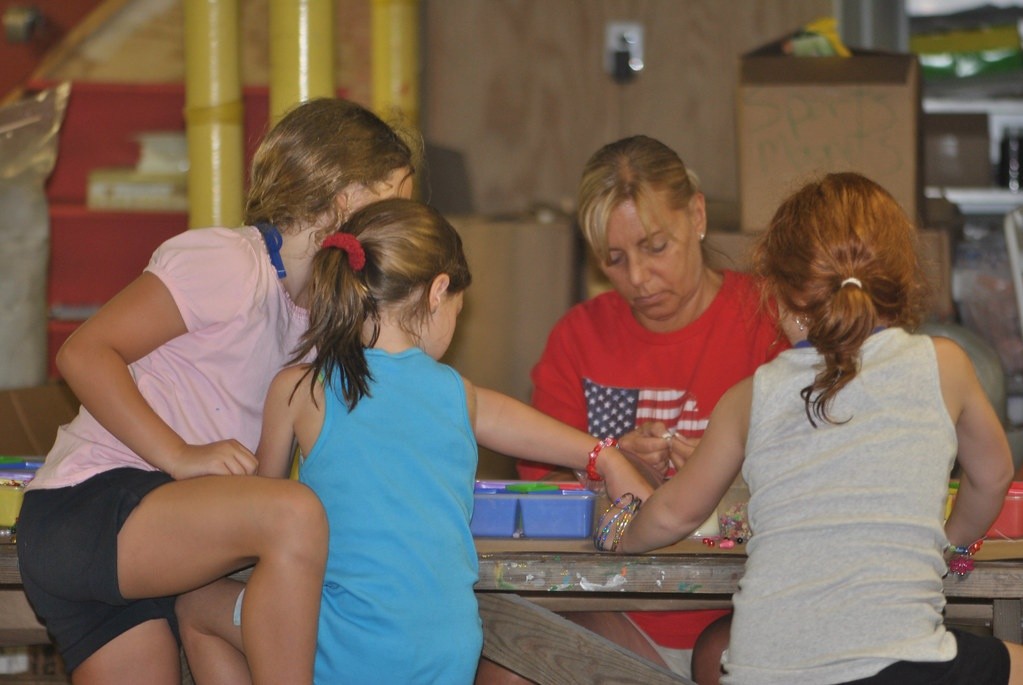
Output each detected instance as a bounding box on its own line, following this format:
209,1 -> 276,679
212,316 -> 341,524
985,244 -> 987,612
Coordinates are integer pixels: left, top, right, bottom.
514,137 -> 796,679
589,170 -> 1023,684
15,94 -> 660,685
175,196 -> 483,685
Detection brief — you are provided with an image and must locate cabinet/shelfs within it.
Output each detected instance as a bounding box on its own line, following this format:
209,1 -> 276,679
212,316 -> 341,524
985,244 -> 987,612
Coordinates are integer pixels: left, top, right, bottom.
835,0 -> 1023,203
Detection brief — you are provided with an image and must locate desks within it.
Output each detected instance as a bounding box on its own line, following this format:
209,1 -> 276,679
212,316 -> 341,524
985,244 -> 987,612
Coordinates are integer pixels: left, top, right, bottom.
1,500 -> 1022,685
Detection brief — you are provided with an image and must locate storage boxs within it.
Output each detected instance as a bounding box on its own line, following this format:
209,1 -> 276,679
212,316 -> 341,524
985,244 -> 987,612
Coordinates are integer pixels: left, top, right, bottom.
445,34 -> 990,544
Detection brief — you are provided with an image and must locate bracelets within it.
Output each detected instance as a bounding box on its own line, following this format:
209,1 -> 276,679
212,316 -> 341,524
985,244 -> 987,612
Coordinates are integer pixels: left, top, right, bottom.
592,491 -> 642,553
940,518 -> 985,578
586,436 -> 621,482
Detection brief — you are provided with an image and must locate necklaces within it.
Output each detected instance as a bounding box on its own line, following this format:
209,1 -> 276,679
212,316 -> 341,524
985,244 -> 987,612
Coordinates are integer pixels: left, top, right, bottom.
794,327 -> 885,349
